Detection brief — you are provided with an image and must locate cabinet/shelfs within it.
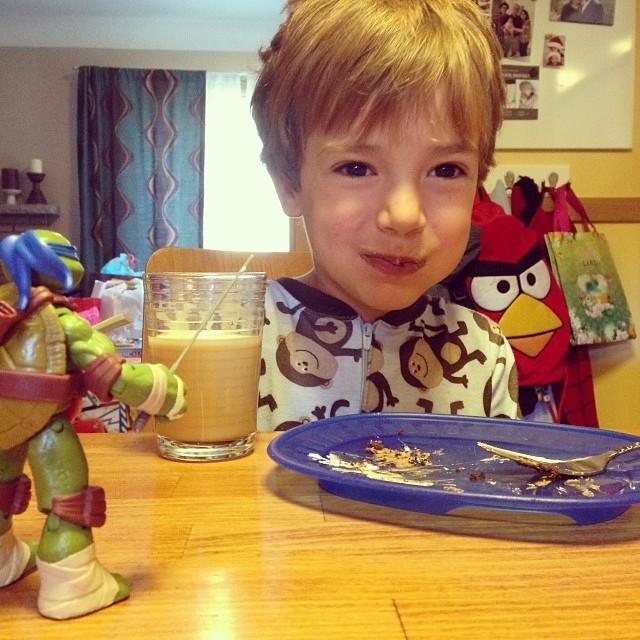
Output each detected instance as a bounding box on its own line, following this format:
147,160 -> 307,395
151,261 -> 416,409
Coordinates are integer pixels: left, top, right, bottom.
2,205 -> 59,233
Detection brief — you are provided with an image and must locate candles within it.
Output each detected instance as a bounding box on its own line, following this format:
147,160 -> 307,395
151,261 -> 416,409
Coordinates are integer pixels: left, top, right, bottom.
2,169 -> 20,190
27,159 -> 43,173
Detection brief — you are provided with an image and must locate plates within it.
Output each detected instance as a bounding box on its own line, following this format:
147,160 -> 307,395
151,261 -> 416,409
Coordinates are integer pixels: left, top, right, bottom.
265,412 -> 640,526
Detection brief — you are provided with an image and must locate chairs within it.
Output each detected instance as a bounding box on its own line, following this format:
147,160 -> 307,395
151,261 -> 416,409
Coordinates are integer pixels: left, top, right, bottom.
139,247 -> 314,431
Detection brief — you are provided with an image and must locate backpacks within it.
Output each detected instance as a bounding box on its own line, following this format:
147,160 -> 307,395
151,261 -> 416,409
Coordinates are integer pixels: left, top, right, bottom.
445,179 -> 572,389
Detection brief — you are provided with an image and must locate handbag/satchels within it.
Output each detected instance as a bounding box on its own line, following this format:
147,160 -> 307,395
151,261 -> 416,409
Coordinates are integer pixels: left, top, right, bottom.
544,180 -> 637,348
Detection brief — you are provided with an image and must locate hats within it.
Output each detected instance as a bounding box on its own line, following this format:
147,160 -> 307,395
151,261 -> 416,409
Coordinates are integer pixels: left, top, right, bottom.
548,36 -> 564,50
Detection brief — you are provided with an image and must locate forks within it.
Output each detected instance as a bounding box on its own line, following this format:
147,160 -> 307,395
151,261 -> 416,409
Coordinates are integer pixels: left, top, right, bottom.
475,440 -> 640,478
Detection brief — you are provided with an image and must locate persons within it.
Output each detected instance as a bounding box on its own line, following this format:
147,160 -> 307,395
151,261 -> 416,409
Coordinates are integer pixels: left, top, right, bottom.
518,80 -> 537,108
545,37 -> 564,66
508,4 -> 523,28
494,2 -> 509,44
502,18 -> 515,39
250,1 -> 523,432
560,0 -> 604,23
515,9 -> 532,56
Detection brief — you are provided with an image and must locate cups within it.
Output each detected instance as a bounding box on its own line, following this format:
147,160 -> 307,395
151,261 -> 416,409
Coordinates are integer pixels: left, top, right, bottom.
141,271 -> 267,461
